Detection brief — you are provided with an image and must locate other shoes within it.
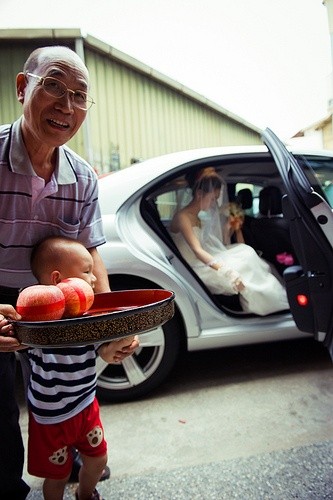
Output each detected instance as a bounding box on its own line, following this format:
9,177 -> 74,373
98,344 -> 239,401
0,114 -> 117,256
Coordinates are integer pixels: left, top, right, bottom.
68,448 -> 110,482
75,487 -> 105,500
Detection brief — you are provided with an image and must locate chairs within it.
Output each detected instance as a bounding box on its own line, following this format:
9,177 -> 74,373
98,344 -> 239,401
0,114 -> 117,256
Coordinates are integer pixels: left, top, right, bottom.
234,188 -> 257,257
254,185 -> 293,279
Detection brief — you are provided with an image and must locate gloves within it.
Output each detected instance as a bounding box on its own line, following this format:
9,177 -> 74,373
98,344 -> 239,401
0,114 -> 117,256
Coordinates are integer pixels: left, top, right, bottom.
218,266 -> 246,291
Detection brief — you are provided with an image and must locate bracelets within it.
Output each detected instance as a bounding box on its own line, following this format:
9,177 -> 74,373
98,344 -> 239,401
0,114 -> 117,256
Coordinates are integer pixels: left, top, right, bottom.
208,259 -> 219,267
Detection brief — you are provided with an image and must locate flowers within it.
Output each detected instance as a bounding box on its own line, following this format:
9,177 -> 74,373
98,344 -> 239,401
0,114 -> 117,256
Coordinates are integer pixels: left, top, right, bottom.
220,203 -> 244,229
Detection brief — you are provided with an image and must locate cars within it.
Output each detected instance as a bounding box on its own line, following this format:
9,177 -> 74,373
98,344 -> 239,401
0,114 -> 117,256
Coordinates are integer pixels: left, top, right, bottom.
90,128 -> 333,400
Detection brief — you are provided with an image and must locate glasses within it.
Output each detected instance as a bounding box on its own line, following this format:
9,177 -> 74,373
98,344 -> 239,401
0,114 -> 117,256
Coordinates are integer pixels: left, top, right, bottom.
27,73 -> 95,111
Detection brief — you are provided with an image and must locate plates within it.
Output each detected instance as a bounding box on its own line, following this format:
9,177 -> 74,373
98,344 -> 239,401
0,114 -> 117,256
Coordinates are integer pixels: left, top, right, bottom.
6,289 -> 175,350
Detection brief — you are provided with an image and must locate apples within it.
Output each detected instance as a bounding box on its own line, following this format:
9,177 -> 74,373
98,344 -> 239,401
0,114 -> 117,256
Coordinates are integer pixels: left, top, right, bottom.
16,285 -> 65,322
56,278 -> 94,319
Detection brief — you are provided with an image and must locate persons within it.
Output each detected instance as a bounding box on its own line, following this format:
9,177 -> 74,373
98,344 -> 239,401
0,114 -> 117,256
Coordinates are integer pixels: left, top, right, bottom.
0,46 -> 140,500
0,235 -> 135,500
170,175 -> 290,316
